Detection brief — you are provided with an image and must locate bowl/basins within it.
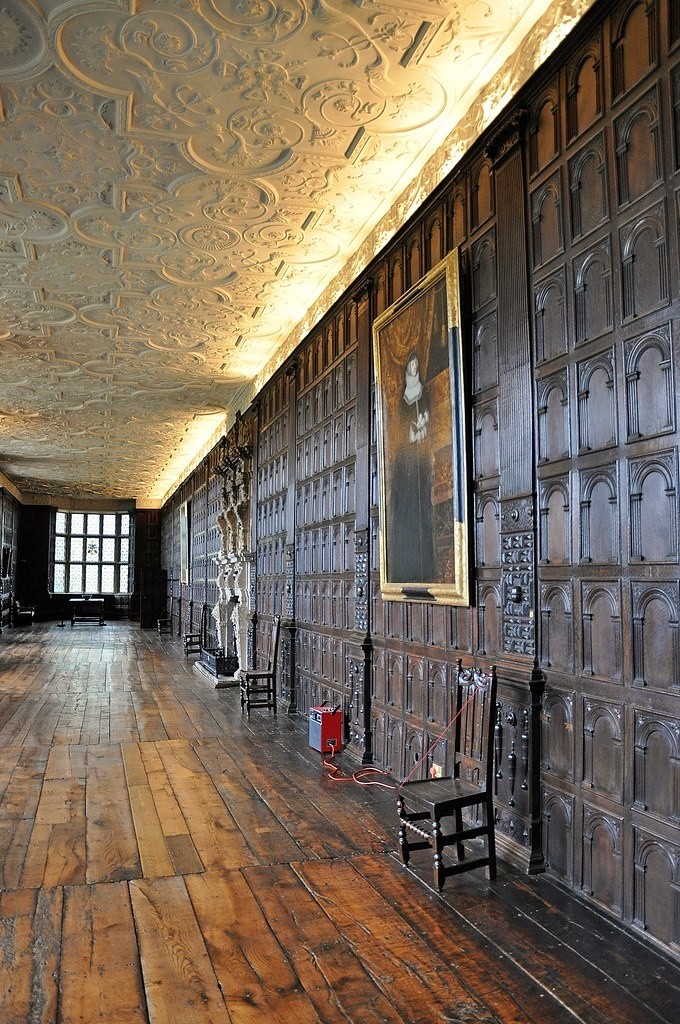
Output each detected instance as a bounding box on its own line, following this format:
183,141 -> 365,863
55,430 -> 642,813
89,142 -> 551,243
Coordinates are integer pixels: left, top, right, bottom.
82,595 -> 92,600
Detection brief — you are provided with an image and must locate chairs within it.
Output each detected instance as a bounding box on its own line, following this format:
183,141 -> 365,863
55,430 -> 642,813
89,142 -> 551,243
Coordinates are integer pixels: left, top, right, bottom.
14,600 -> 36,625
397,658 -> 498,892
157,597 -> 173,637
182,604 -> 204,658
240,614 -> 281,716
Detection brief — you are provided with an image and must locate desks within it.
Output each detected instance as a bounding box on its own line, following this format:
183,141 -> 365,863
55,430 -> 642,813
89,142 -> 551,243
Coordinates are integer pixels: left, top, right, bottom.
69,599 -> 105,626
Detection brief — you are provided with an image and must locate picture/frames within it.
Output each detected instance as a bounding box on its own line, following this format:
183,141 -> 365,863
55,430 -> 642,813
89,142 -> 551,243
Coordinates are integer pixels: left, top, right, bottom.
371,247 -> 472,608
